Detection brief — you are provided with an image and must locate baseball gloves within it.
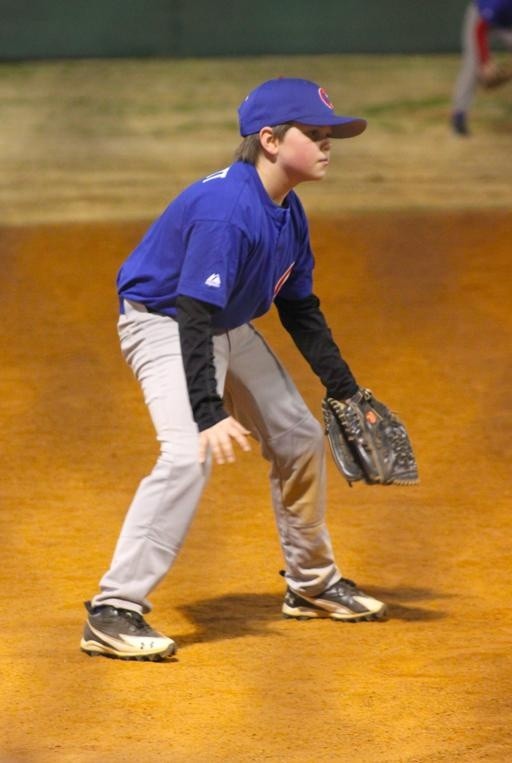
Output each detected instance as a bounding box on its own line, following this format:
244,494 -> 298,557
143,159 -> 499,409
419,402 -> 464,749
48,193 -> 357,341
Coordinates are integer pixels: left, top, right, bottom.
322,387 -> 417,486
478,56 -> 512,86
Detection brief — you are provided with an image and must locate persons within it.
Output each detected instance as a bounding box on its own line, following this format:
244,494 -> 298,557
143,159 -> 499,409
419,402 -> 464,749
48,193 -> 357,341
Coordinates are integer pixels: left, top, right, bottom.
447,2 -> 512,141
75,73 -> 393,663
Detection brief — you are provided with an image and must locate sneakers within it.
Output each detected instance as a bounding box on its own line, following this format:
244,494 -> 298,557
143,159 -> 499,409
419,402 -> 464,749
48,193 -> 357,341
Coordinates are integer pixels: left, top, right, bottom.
278,569 -> 390,624
450,114 -> 468,135
81,598 -> 177,661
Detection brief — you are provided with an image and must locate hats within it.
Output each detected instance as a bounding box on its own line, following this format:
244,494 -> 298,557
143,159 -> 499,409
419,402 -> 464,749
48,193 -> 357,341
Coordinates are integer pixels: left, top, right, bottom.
239,75 -> 367,138
